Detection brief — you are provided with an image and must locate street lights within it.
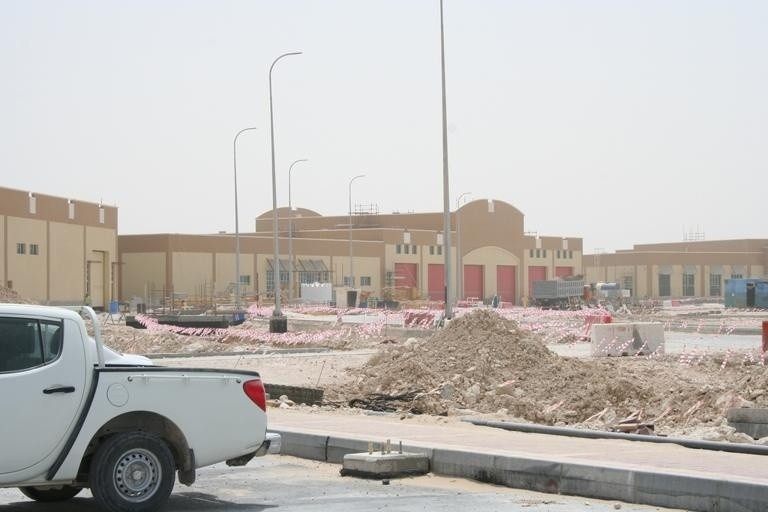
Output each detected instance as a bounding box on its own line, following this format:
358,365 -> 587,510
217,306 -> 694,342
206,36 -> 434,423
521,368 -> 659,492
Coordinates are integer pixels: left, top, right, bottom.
348,173 -> 366,290
268,51 -> 302,333
457,191 -> 472,301
288,158 -> 309,305
233,126 -> 257,310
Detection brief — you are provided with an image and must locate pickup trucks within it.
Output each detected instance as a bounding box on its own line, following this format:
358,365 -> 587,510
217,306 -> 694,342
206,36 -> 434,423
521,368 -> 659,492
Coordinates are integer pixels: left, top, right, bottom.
1,303 -> 281,511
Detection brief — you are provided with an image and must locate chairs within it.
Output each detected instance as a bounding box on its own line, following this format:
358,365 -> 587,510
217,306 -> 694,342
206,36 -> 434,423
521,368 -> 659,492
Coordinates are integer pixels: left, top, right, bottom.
35,328 -> 61,367
7,326 -> 35,370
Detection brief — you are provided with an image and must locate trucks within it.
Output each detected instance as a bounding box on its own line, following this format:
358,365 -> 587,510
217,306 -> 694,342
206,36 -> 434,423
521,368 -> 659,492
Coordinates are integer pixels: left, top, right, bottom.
533,279 -> 593,311
